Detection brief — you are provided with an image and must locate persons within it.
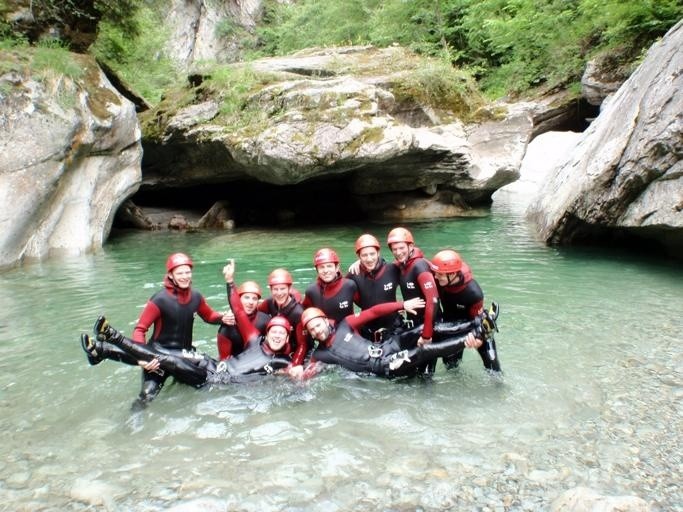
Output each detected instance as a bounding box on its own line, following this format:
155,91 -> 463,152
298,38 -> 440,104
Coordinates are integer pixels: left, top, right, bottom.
220,268 -> 307,376
343,234 -> 410,342
301,247 -> 363,338
347,226 -> 441,380
79,255 -> 305,388
216,280 -> 273,364
289,296 -> 500,378
129,253 -> 222,411
425,249 -> 503,375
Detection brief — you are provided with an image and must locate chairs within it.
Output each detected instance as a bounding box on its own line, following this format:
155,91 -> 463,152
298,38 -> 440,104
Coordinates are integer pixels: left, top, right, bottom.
475,301 -> 499,328
471,310 -> 495,345
93,316 -> 117,342
81,334 -> 104,365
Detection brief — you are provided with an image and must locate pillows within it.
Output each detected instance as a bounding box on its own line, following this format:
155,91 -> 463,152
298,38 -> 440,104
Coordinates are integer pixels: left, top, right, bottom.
167,253 -> 192,273
238,282 -> 261,299
431,251 -> 461,274
269,270 -> 292,287
301,307 -> 327,330
314,249 -> 339,267
355,235 -> 380,253
266,316 -> 290,335
387,228 -> 414,246
